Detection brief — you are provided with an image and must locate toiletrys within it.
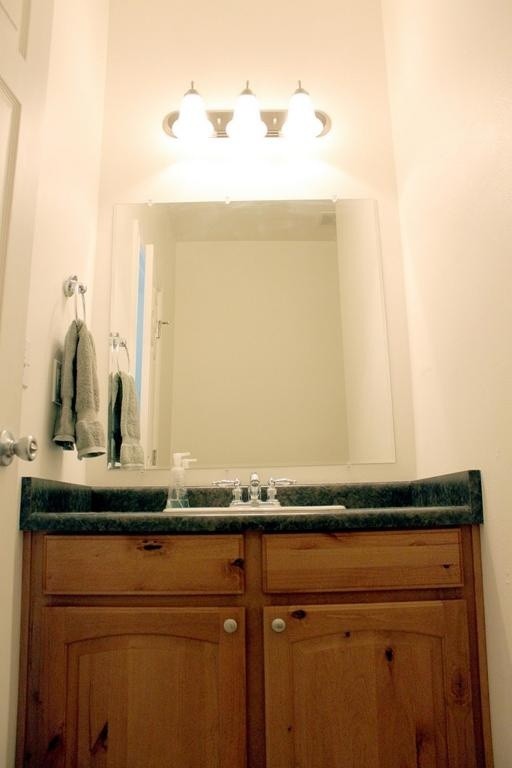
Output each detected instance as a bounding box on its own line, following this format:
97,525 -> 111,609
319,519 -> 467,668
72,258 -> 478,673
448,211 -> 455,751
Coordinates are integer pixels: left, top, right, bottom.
183,458 -> 197,476
166,451 -> 190,507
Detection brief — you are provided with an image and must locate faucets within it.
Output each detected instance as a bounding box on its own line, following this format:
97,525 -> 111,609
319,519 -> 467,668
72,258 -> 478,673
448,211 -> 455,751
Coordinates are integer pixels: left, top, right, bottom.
247,473 -> 264,502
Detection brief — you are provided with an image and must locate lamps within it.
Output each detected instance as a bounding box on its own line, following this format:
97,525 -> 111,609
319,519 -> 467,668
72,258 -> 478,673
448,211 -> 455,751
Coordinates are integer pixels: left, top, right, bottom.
162,81 -> 331,146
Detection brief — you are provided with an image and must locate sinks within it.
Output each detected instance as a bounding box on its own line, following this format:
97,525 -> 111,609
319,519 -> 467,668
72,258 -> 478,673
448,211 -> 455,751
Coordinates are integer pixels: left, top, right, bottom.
161,504 -> 346,513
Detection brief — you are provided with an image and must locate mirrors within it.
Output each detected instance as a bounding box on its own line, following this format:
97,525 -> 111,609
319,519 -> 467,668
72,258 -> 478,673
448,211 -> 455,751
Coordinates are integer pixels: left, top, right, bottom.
107,199 -> 396,472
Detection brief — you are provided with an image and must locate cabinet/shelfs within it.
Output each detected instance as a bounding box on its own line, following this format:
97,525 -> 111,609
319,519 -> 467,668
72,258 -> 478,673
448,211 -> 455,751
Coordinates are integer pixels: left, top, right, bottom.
15,524 -> 495,768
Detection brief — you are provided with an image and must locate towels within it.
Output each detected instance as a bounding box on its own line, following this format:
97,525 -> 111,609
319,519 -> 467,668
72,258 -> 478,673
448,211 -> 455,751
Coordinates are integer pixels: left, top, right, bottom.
109,372 -> 145,471
52,319 -> 106,460
